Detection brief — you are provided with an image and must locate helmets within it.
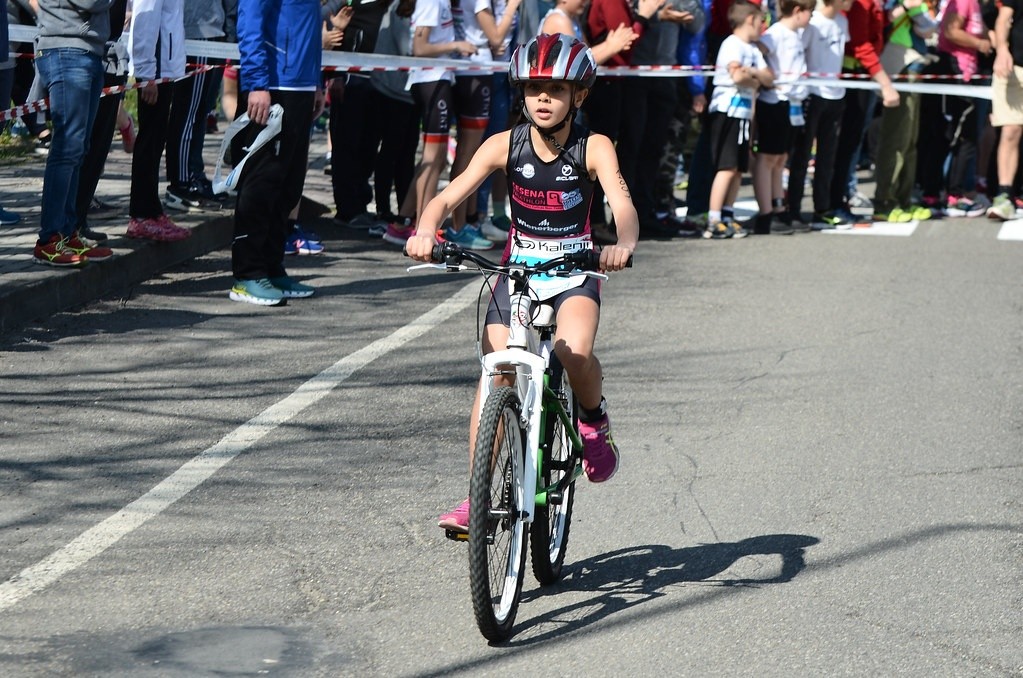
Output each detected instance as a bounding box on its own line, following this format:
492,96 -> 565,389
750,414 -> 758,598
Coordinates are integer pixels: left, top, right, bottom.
507,32 -> 599,89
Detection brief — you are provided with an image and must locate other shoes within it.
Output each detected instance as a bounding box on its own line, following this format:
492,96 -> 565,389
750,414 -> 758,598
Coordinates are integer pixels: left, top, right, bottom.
853,166 -> 875,182
806,162 -> 817,174
207,112 -> 218,133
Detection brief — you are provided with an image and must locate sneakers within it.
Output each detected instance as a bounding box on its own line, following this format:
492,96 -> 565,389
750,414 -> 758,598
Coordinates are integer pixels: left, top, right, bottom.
369,215 -> 513,251
119,116 -> 137,155
575,415 -> 620,483
228,275 -> 287,306
674,181 -> 689,190
269,275 -> 316,298
125,212 -> 192,242
163,171 -> 229,212
33,132 -> 53,156
276,221 -> 326,255
0,205 -> 19,224
73,222 -> 108,248
66,229 -> 113,262
872,201 -> 932,223
637,194 -> 873,238
437,493 -> 493,534
917,178 -> 1023,220
86,195 -> 124,219
331,209 -> 374,230
31,233 -> 90,268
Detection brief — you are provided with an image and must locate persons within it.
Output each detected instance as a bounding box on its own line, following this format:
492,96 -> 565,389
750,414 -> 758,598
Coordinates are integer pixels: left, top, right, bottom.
0,0 -> 1023,306
407,33 -> 639,531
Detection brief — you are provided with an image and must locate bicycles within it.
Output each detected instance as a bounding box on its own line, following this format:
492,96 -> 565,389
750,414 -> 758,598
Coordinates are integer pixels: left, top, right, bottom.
403,240 -> 635,641
